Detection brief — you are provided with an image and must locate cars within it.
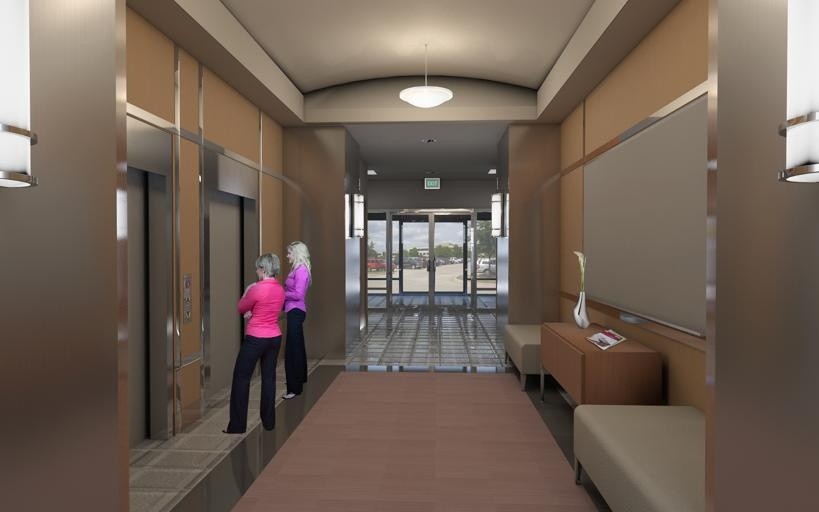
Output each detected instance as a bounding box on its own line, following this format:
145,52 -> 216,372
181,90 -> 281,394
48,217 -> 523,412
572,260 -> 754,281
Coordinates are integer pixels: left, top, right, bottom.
397,257 -> 422,269
477,258 -> 496,277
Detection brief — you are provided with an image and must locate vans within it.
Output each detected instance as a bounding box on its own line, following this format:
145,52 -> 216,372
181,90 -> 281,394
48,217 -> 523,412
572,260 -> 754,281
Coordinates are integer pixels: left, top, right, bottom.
368,258 -> 396,272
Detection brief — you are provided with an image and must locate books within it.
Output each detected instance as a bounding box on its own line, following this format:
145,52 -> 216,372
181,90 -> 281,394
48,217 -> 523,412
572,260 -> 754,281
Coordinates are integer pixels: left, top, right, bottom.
586,329 -> 627,350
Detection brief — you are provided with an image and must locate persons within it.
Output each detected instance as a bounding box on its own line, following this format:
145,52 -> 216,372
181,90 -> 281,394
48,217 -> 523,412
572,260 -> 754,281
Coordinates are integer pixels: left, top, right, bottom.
279,241 -> 314,401
220,252 -> 287,434
